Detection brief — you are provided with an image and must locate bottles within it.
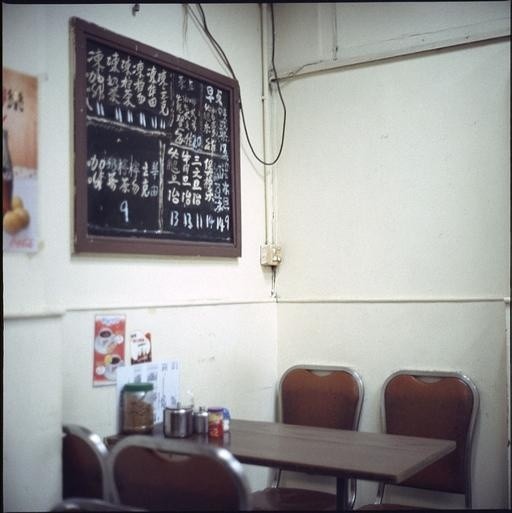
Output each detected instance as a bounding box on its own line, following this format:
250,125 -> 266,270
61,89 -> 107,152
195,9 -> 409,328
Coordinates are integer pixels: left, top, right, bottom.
163,406 -> 224,439
124,382 -> 154,434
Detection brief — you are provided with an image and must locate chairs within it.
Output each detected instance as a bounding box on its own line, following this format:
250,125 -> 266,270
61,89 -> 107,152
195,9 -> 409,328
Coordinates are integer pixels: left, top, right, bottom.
109,434 -> 251,512
61,423 -> 113,504
352,369 -> 480,512
251,363 -> 365,513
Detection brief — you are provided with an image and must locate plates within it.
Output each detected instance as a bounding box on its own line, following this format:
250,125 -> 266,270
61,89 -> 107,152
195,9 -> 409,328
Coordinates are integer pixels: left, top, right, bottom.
104,361 -> 122,379
96,337 -> 117,353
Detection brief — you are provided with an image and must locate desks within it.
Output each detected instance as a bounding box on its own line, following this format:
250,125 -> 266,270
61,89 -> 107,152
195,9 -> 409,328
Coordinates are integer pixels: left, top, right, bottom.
109,414 -> 458,513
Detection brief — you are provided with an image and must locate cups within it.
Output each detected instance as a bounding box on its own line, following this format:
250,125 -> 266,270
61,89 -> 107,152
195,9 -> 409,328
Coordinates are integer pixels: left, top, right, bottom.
108,354 -> 124,369
98,328 -> 114,345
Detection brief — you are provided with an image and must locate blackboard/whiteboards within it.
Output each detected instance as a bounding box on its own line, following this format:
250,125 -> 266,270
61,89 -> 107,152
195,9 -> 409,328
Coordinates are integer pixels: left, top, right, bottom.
69,16 -> 242,258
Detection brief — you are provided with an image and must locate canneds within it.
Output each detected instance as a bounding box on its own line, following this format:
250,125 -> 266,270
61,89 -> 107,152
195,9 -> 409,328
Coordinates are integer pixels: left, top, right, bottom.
208,407 -> 224,438
